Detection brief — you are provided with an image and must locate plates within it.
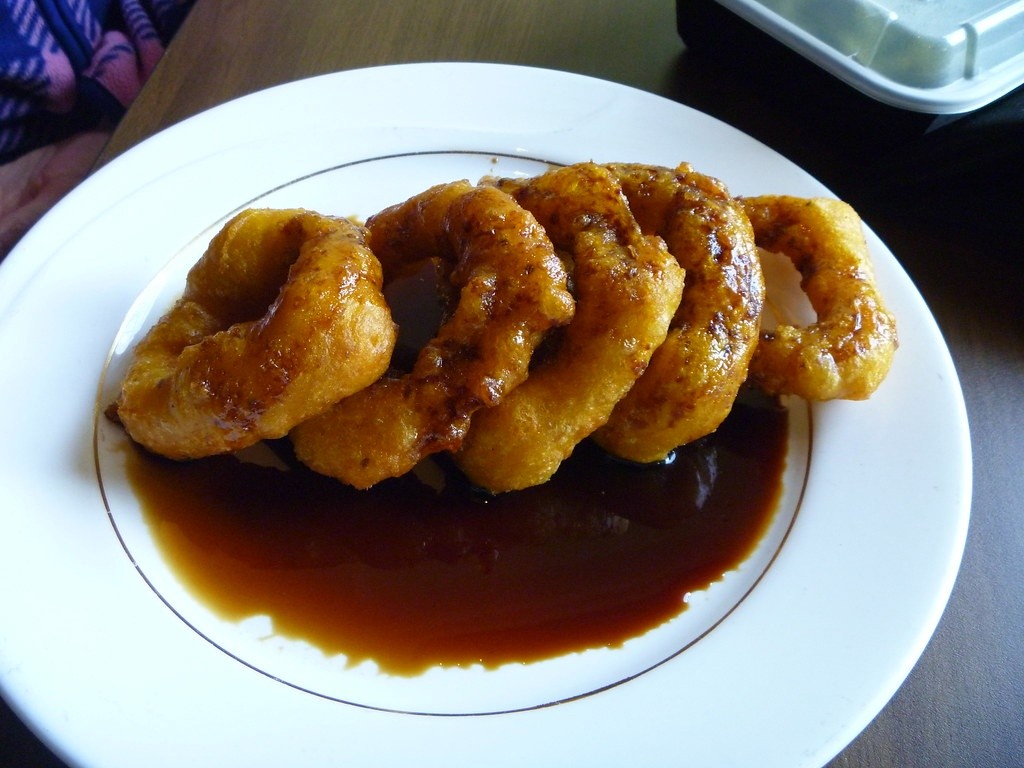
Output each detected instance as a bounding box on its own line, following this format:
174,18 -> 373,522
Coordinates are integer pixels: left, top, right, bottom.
0,60 -> 975,768
